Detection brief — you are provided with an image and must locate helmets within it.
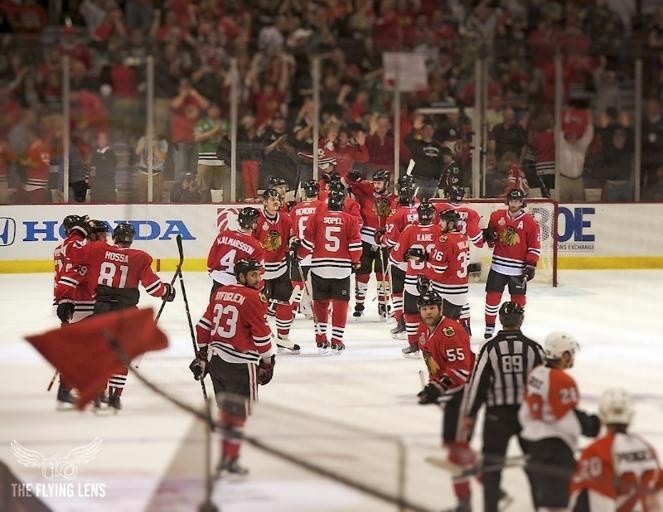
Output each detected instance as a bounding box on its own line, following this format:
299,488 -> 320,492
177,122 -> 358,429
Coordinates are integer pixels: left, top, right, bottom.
234,259 -> 262,282
65,215 -> 134,243
236,170 -> 525,231
418,292 -> 635,426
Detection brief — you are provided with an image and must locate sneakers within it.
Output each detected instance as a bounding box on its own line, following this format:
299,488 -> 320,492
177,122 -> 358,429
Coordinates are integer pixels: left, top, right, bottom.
217,457 -> 249,476
275,302 -> 496,353
58,384 -> 121,409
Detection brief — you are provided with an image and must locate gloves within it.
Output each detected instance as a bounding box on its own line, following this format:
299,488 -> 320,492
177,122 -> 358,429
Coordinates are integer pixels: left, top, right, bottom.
57,302 -> 76,322
190,357 -> 208,381
162,282 -> 177,302
521,267 -> 536,281
258,355 -> 276,385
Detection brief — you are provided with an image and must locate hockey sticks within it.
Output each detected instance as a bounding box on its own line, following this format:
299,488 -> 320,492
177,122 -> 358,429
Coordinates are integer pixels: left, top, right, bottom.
177,267 -> 243,440
373,199 -> 388,323
153,235 -> 183,327
405,159 -> 416,226
511,274 -> 529,289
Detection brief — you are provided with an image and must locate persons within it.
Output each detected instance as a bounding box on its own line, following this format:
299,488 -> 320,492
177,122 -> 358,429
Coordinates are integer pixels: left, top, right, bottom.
0,0 -> 663,202
53,215 -> 176,411
189,257 -> 279,476
517,330 -> 599,511
464,301 -> 546,511
485,188 -> 540,339
208,169 -> 485,355
566,388 -> 663,512
417,290 -> 514,512
